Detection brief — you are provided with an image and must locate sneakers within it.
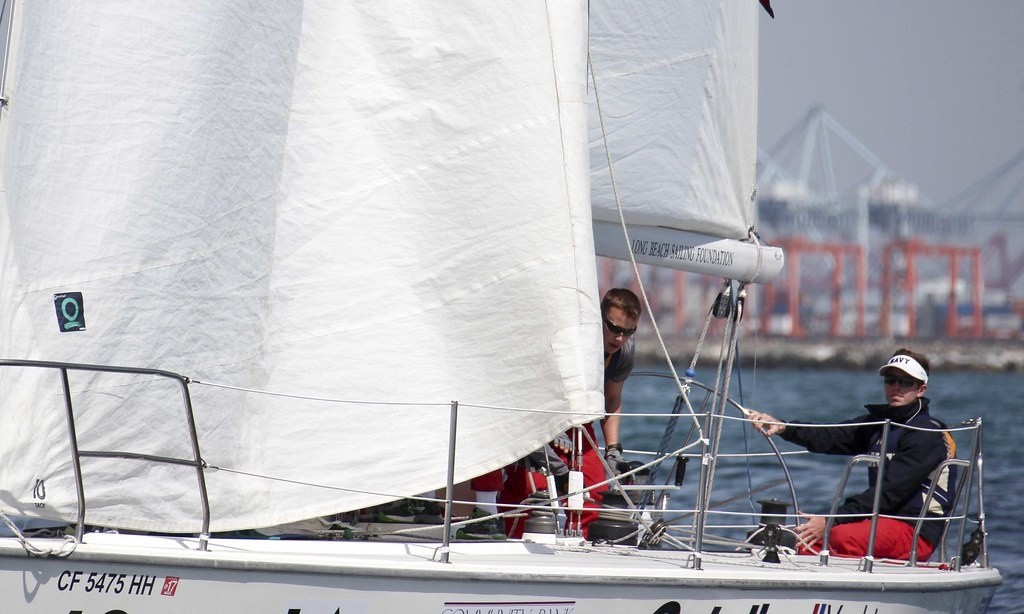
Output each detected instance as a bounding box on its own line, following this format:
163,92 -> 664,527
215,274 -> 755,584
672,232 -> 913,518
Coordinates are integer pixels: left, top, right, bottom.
457,507 -> 507,540
377,496 -> 444,524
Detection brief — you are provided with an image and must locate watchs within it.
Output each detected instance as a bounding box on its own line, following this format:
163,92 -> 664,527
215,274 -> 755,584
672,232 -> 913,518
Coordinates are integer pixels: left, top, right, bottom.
605,443 -> 623,453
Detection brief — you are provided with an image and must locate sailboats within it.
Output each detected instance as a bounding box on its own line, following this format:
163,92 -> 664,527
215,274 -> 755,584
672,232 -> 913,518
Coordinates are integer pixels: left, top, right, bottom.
0,0 -> 1004,614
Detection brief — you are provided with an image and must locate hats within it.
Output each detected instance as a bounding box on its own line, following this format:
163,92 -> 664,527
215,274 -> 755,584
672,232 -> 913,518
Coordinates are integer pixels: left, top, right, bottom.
877,354 -> 930,384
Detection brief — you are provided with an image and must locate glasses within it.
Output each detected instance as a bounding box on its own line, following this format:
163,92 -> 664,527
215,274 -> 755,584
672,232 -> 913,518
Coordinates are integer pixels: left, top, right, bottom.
603,316 -> 638,337
883,373 -> 915,387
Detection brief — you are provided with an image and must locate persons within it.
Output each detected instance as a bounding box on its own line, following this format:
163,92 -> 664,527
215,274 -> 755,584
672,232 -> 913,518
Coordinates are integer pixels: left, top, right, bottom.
376,288 -> 642,541
748,351 -> 957,560
